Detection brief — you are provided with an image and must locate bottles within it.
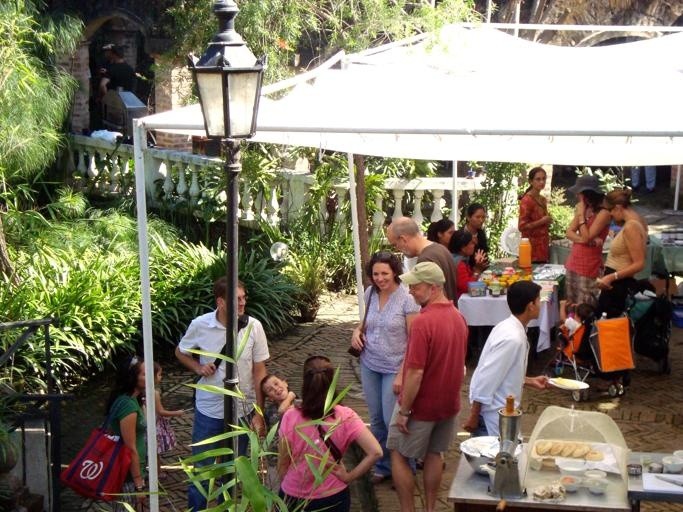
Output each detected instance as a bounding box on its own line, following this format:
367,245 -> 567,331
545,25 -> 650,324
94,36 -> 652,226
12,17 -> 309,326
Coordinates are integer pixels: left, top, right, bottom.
599,312 -> 607,320
519,237 -> 531,273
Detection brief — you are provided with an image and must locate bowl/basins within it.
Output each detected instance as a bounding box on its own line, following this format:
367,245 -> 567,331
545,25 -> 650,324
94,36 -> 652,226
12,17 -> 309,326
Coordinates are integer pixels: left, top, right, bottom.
558,461 -> 586,476
459,435 -> 522,475
627,464 -> 642,476
662,456 -> 682,472
672,450 -> 683,460
590,480 -> 608,495
559,476 -> 580,493
585,470 -> 607,481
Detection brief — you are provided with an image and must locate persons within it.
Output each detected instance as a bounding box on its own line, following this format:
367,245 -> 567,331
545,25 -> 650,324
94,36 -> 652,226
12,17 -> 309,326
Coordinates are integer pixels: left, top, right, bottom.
518,166 -> 648,385
278,355 -> 384,511
99,43 -> 114,73
260,374 -> 299,493
144,361 -> 186,479
102,356 -> 146,512
428,204 -> 492,306
459,280 -> 550,441
100,46 -> 136,95
175,276 -> 271,512
351,217 -> 469,511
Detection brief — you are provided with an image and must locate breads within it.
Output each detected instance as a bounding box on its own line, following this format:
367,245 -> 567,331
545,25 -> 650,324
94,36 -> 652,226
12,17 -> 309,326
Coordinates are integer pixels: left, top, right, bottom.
537,440 -> 604,461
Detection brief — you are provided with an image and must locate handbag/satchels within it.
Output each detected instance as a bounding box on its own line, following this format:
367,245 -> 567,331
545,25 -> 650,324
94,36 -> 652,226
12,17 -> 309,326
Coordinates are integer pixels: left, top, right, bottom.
60,428 -> 132,501
347,337 -> 365,358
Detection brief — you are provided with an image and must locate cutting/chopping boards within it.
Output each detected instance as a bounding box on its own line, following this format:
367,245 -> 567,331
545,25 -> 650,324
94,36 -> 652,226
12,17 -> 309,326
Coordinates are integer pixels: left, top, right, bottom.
642,472 -> 683,495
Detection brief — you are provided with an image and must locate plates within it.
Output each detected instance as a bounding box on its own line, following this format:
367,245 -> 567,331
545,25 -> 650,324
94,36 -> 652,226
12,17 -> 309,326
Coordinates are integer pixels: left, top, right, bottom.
547,378 -> 590,391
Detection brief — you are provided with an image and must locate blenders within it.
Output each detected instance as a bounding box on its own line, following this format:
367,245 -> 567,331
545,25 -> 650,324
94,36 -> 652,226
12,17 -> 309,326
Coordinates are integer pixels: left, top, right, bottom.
487,395 -> 529,500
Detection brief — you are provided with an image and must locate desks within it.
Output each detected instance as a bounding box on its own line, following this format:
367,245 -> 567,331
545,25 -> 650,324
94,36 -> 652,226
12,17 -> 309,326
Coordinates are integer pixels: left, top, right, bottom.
627,452 -> 683,511
551,234 -> 660,280
659,238 -> 683,278
447,437 -> 631,512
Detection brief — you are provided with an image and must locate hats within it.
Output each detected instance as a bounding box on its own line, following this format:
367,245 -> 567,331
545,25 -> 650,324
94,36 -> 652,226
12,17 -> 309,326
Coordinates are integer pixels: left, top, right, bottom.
568,176 -> 605,195
398,261 -> 446,286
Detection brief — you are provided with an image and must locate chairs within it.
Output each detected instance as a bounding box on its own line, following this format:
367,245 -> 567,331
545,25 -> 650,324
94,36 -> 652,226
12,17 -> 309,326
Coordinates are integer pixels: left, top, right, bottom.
661,229 -> 683,239
501,227 -> 521,258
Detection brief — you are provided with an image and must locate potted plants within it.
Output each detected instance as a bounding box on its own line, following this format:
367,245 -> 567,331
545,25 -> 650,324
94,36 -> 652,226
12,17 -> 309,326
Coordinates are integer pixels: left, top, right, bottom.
467,161 -> 477,178
281,253 -> 327,322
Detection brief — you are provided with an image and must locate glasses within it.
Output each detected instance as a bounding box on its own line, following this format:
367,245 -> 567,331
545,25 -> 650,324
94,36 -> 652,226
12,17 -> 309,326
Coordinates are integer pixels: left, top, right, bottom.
608,206 -> 617,211
372,251 -> 393,261
129,355 -> 138,370
533,177 -> 546,181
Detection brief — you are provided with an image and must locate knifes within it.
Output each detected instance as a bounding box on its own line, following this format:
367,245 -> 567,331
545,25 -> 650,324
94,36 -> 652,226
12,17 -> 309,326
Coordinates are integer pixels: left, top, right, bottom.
655,474 -> 683,489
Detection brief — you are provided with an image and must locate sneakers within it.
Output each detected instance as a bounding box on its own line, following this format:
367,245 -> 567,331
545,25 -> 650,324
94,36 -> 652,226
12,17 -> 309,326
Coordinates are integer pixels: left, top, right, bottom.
369,472 -> 384,485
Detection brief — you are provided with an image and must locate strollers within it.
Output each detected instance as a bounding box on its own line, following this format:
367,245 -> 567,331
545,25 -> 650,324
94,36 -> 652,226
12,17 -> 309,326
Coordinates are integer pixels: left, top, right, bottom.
607,273 -> 676,375
542,299 -> 639,399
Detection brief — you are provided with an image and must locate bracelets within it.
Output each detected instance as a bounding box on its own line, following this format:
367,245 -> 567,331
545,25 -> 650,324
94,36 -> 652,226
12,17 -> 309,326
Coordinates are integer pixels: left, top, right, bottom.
472,272 -> 479,276
132,474 -> 142,479
135,485 -> 144,492
